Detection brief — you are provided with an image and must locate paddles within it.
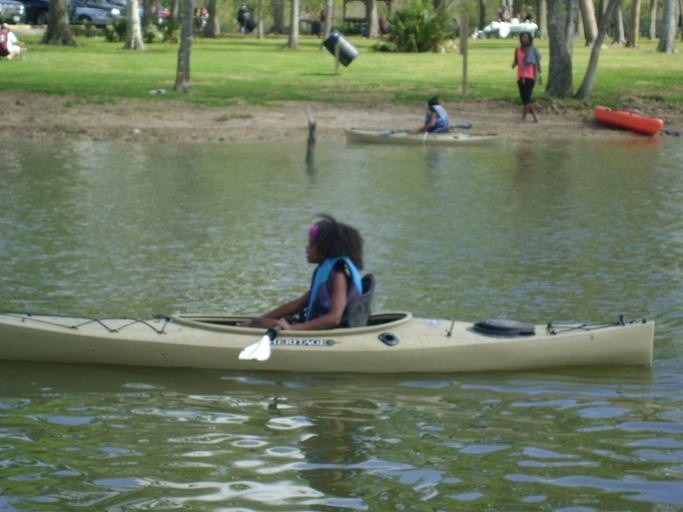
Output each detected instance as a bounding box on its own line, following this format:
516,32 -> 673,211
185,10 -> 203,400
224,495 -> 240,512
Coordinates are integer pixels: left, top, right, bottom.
238,326 -> 282,361
376,122 -> 473,139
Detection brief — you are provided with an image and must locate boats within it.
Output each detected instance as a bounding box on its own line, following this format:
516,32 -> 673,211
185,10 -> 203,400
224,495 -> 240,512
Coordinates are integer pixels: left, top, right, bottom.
593,104 -> 666,137
342,124 -> 500,146
0,306 -> 655,375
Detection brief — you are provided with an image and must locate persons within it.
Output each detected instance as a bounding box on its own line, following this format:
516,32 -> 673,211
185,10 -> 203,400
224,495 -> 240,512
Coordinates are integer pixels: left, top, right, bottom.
233,216 -> 363,331
510,31 -> 541,127
158,2 -> 325,39
406,95 -> 449,135
0,23 -> 24,60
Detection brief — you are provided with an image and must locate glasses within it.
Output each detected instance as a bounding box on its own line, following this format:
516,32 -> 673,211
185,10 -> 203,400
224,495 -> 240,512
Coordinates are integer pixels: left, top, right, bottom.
311,222 -> 323,238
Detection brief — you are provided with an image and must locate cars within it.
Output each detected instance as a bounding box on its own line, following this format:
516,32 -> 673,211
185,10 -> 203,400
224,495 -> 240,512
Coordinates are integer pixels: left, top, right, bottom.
0,0 -> 181,31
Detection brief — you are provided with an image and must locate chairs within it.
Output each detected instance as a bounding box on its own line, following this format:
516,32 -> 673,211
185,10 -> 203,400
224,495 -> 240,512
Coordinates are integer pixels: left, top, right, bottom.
345,271 -> 375,327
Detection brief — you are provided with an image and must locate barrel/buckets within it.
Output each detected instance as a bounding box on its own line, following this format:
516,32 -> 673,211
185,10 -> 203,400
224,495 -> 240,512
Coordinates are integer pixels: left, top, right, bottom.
320,29 -> 359,69
